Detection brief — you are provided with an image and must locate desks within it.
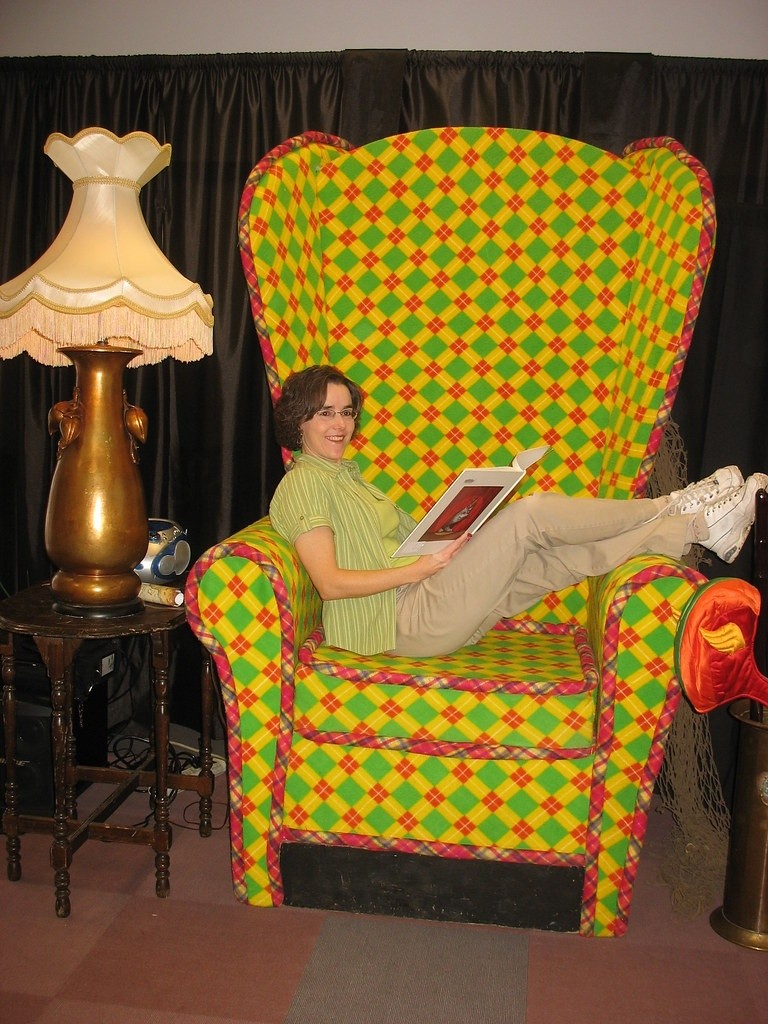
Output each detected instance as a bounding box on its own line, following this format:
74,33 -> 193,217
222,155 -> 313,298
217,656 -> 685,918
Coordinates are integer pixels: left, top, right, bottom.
0,581 -> 220,918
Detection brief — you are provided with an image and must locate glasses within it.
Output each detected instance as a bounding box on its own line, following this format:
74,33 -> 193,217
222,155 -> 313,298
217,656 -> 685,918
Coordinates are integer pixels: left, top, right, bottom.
311,409 -> 357,421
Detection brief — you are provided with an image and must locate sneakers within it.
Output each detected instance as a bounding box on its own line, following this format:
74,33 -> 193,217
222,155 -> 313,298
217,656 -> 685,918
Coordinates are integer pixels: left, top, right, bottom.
670,465 -> 745,515
695,472 -> 768,563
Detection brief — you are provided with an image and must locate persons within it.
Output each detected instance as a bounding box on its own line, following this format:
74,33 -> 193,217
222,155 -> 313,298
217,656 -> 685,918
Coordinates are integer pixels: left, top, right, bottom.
268,366 -> 768,659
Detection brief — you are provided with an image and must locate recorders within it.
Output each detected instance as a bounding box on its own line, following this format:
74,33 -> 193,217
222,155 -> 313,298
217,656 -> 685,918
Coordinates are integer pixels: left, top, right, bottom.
132,518 -> 192,585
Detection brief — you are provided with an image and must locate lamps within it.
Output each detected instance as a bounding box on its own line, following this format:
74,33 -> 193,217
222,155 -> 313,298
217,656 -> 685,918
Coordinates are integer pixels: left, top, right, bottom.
0,127 -> 214,620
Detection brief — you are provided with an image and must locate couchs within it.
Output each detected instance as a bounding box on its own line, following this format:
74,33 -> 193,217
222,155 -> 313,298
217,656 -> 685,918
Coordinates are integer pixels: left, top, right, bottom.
180,127 -> 720,937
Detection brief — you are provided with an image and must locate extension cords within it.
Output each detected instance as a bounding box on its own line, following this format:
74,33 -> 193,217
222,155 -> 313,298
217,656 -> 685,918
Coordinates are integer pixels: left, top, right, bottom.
148,758 -> 227,798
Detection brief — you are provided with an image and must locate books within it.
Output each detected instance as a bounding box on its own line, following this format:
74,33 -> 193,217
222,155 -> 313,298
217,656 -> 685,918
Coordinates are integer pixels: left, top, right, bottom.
390,444 -> 551,558
